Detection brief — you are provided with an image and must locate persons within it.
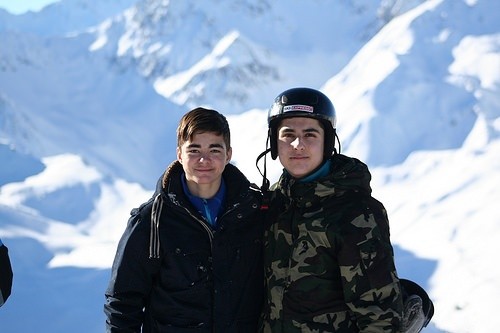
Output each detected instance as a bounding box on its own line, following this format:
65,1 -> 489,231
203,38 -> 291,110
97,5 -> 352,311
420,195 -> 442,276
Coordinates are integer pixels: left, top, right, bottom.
104,107 -> 269,333
262,88 -> 406,333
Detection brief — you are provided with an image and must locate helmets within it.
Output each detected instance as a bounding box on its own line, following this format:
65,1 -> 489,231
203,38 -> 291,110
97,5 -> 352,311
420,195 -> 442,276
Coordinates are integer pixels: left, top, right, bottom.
267,88 -> 335,160
